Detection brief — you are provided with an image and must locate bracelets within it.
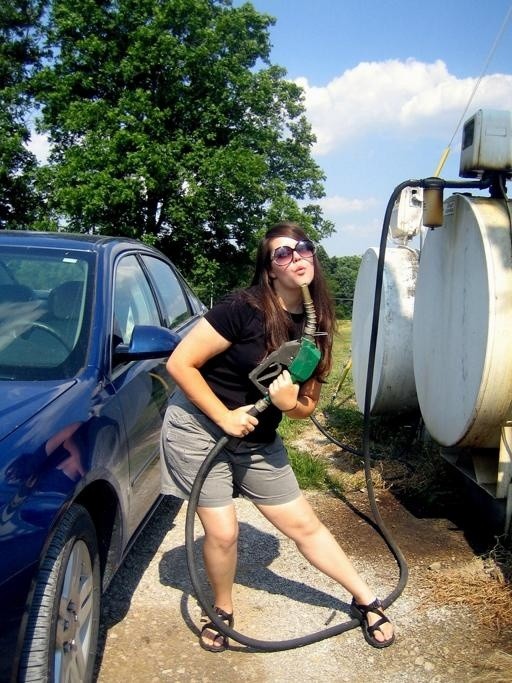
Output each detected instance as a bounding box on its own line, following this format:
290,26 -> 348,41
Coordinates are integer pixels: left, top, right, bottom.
280,403 -> 297,411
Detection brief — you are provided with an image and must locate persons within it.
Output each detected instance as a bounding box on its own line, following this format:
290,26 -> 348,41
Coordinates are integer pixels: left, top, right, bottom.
161,220 -> 394,652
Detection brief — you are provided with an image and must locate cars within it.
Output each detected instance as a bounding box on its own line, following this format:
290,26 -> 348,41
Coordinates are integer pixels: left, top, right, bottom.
0,229 -> 215,683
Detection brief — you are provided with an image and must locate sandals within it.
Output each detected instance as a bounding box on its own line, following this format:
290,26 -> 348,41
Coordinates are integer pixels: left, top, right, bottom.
350,597 -> 395,649
199,606 -> 234,653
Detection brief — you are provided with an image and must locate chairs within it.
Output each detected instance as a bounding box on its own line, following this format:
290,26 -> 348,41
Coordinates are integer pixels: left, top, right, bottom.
27,281 -> 84,351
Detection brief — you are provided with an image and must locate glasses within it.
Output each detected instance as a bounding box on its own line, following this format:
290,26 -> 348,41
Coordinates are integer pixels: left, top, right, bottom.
270,239 -> 317,266
